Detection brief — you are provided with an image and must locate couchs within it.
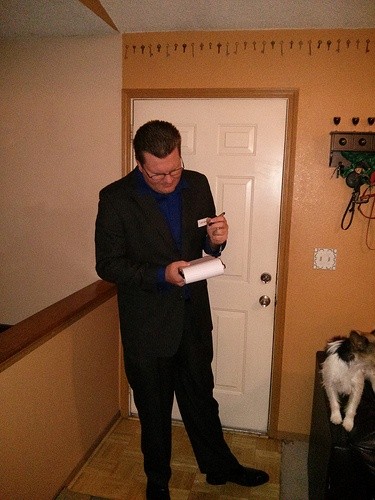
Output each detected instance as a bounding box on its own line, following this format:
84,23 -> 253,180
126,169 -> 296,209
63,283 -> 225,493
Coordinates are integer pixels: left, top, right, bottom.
308,351 -> 375,500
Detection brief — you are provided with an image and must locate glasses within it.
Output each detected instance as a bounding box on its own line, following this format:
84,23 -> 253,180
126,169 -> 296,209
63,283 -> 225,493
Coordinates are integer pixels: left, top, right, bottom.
141,157 -> 185,180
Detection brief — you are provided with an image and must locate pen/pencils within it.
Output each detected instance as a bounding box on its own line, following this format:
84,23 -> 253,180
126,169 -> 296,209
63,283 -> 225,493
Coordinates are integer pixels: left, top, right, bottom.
209,211 -> 225,227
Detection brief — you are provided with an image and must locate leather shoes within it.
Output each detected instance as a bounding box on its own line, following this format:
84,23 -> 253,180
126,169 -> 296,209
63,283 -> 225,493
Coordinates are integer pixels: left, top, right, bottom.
206,465 -> 269,487
145,477 -> 170,500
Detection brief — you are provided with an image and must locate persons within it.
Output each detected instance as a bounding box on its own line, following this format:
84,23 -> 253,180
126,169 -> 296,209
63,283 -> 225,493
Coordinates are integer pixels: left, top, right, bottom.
94,120 -> 269,500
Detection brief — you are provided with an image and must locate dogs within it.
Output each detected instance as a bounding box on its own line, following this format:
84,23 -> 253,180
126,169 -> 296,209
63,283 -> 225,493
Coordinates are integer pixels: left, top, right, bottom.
317,329 -> 375,432
